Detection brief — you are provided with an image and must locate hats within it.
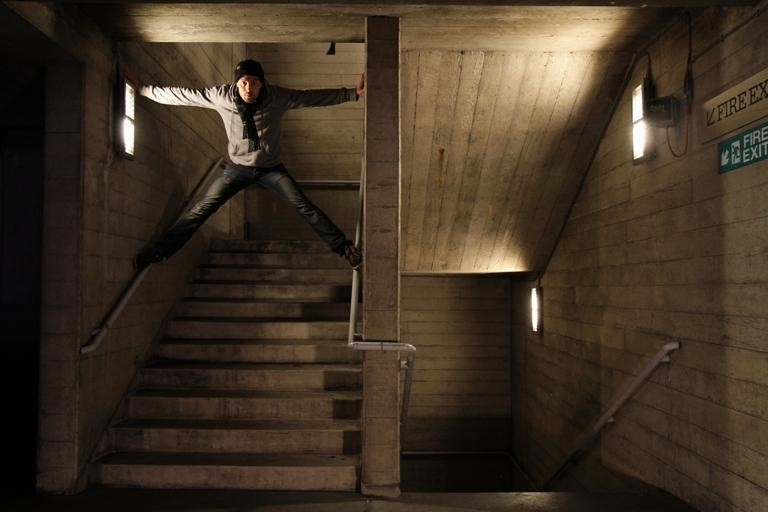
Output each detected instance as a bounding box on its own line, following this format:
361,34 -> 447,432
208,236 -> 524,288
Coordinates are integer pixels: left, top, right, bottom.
234,60 -> 264,84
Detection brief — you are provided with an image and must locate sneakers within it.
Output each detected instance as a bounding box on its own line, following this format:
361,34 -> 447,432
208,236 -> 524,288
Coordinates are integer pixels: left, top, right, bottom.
344,244 -> 362,267
135,244 -> 163,269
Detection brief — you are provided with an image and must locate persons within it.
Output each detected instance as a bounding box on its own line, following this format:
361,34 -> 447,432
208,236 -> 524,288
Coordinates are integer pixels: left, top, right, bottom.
118,58 -> 365,271
